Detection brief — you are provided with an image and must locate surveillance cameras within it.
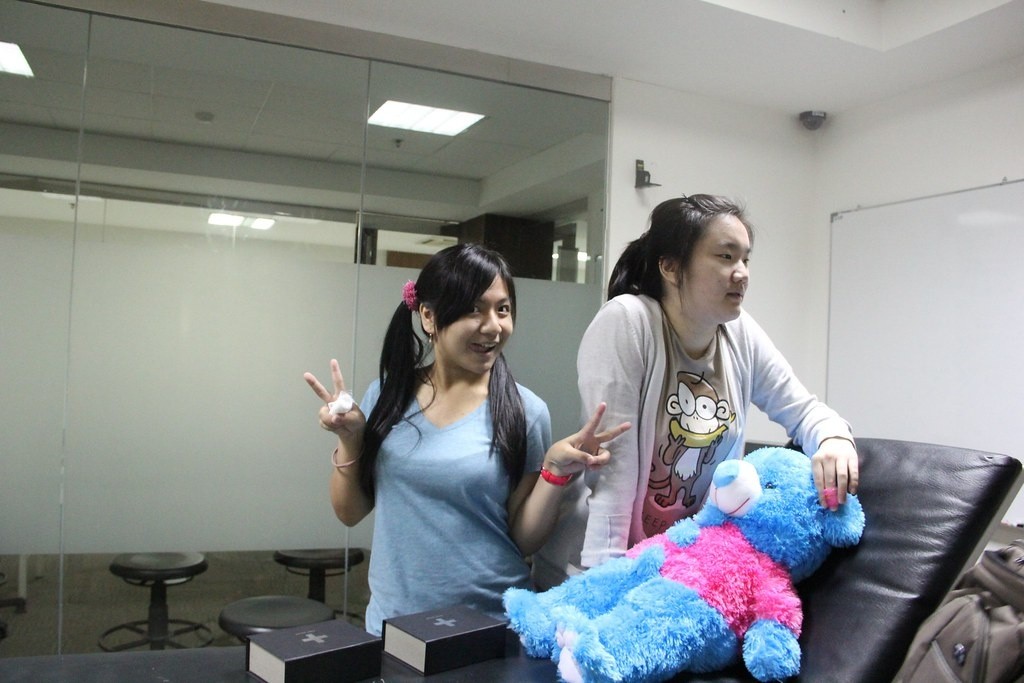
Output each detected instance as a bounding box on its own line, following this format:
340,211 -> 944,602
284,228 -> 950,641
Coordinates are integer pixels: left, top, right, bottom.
799,111 -> 826,130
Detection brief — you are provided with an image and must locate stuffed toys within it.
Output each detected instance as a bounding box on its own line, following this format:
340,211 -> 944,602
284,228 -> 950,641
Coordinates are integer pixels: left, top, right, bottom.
503,447 -> 864,683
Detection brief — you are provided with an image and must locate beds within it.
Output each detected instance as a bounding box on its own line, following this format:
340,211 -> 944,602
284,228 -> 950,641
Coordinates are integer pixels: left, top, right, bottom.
0,434 -> 1024,682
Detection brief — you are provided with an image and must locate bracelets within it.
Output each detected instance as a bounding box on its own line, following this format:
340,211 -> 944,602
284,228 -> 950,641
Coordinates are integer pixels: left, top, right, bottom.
541,465 -> 573,485
332,444 -> 366,467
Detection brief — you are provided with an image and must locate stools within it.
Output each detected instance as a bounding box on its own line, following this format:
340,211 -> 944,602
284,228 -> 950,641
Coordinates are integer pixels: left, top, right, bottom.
219,594 -> 335,647
98,550 -> 214,655
273,549 -> 367,628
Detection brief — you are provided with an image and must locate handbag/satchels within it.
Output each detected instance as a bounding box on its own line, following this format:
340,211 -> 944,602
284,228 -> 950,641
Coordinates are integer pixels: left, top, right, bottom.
897,538 -> 1024,683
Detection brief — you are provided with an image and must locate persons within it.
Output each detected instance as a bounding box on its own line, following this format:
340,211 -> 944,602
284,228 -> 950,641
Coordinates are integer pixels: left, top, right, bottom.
528,194 -> 861,591
303,242 -> 631,637
